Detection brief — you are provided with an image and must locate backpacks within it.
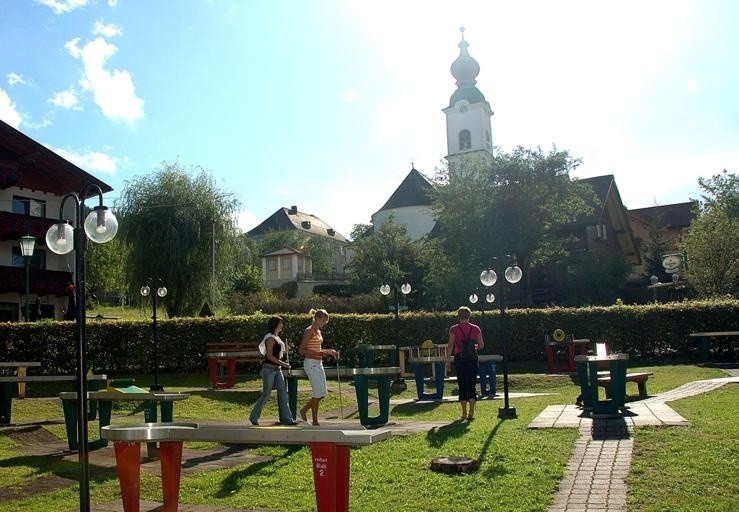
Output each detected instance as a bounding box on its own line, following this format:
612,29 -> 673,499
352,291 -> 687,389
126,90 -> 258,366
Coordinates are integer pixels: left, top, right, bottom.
456,325 -> 478,362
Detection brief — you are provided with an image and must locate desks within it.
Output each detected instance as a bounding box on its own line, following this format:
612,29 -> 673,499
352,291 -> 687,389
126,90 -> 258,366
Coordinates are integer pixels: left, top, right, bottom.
398,330 -> 739,417
0,341 -> 398,512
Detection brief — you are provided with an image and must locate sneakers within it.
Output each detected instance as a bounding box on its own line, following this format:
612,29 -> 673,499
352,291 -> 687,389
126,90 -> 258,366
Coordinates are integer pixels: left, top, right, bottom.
248,417 -> 298,425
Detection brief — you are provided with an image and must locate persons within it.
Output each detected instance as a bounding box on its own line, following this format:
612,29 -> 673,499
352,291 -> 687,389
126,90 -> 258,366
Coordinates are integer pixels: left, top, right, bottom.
298,308 -> 337,426
445,305 -> 486,421
248,316 -> 298,427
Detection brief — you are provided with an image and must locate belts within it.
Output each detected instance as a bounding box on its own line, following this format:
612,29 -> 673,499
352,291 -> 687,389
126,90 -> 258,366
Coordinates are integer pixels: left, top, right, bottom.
263,363 -> 281,369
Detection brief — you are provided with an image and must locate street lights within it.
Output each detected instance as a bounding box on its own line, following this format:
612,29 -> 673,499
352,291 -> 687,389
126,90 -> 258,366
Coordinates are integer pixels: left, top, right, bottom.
19,232 -> 35,320
467,287 -> 496,336
46,181 -> 119,510
479,252 -> 525,419
141,276 -> 168,392
380,275 -> 414,390
